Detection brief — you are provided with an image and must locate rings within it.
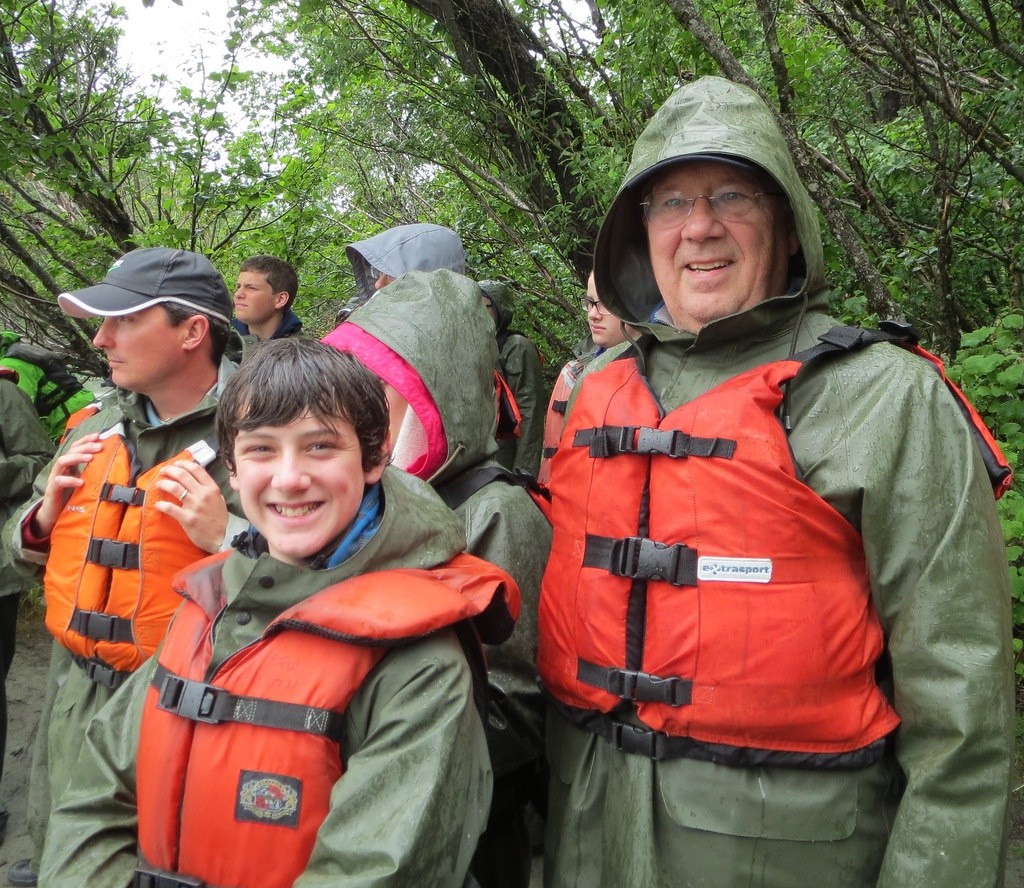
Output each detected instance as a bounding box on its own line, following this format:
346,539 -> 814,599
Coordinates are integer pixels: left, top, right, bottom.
179,490 -> 188,501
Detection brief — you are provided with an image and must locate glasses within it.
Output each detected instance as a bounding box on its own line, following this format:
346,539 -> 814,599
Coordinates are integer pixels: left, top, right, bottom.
370,266 -> 392,282
640,184 -> 781,229
582,298 -> 612,316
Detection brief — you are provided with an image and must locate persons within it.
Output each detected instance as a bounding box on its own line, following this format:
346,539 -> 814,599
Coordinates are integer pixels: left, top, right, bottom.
0,332 -> 96,846
479,279 -> 534,471
538,271 -> 639,489
320,270 -> 554,887
229,256 -> 302,356
336,224 -> 465,325
36,337 -> 521,887
0,248 -> 249,886
536,76 -> 1016,888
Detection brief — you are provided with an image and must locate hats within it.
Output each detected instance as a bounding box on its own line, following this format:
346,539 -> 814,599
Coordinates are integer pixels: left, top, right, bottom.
57,248 -> 233,324
